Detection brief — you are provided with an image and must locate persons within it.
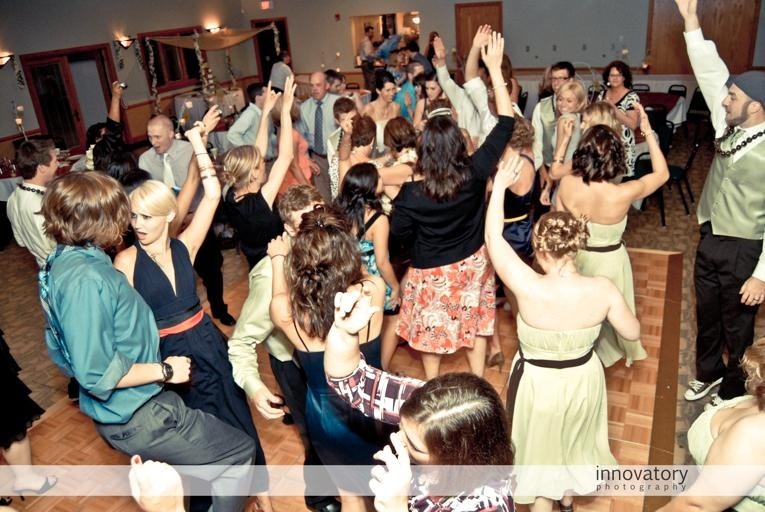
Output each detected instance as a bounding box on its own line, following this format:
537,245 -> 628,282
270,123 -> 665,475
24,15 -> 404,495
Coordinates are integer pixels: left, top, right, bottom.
119,169 -> 155,248
112,120 -> 275,512
416,69 -> 457,134
395,63 -> 422,123
599,59 -> 639,183
1,325 -> 57,506
271,96 -> 319,225
332,162 -> 404,381
93,78 -> 138,181
66,122 -> 107,175
542,83 -> 590,205
222,74 -> 294,270
327,98 -> 365,197
137,116 -> 237,328
360,24 -> 380,94
341,114 -> 374,182
324,284 -> 514,512
7,141 -> 60,270
37,172 -> 257,512
556,102 -> 671,367
324,69 -> 342,96
485,151 -> 641,512
269,50 -> 294,95
432,23 -> 521,155
227,185 -> 345,512
368,74 -> 406,158
494,118 -> 541,308
383,117 -> 420,218
530,61 -> 597,218
229,81 -> 279,188
128,455 -> 187,512
389,30 -> 512,383
266,207 -> 384,512
675,0 -> 765,409
582,103 -> 625,147
654,335 -> 765,512
297,71 -> 344,212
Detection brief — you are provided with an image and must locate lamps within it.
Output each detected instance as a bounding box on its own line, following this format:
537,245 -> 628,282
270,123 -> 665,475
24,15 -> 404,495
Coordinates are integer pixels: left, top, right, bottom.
14,103 -> 30,141
0,56 -> 12,68
118,40 -> 136,49
206,26 -> 222,34
211,31 -> 216,32
206,27 -> 222,35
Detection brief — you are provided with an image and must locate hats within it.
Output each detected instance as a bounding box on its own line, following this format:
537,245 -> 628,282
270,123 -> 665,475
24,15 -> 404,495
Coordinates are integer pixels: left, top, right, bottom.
733,70 -> 765,110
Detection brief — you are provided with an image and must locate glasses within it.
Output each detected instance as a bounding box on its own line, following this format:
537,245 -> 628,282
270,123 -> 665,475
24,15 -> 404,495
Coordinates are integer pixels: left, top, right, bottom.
550,76 -> 572,82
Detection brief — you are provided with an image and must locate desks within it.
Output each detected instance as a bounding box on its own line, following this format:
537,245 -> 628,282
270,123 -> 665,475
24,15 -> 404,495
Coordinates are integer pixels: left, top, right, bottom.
173,86 -> 246,133
1,174 -> 23,251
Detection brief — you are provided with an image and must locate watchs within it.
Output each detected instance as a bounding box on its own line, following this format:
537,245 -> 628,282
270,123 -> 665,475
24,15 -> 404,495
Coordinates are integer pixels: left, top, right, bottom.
155,360 -> 174,384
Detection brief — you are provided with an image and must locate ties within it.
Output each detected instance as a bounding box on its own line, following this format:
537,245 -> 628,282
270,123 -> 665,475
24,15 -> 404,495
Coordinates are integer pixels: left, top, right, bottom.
314,100 -> 325,153
162,154 -> 176,189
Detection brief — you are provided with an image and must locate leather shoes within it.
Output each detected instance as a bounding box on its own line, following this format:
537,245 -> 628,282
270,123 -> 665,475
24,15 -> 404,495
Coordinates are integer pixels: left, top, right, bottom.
216,311 -> 238,327
313,501 -> 342,512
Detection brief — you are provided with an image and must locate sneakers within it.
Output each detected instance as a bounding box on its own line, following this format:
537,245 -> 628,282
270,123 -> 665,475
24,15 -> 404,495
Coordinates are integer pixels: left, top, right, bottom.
685,376 -> 725,400
704,392 -> 725,410
558,502 -> 574,512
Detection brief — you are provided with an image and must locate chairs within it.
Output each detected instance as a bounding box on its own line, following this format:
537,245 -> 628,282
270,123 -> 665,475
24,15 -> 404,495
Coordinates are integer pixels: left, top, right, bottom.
586,82 -> 604,102
518,91 -> 528,111
345,81 -> 361,91
638,142 -> 699,218
295,72 -> 311,101
645,103 -> 679,152
630,81 -> 651,94
516,83 -> 521,98
665,82 -> 687,140
636,151 -> 666,227
687,86 -> 715,143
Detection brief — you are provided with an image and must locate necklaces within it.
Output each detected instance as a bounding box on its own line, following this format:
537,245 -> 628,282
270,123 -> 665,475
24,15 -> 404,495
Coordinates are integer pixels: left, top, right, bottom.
714,126 -> 765,158
16,182 -> 45,196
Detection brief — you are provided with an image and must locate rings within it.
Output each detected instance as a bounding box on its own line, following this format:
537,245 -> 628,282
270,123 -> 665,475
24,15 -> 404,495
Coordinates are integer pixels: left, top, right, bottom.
201,124 -> 207,128
513,169 -> 519,175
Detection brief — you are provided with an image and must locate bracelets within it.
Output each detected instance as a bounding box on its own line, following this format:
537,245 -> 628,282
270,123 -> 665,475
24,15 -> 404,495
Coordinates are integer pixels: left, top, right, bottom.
551,160 -> 564,163
195,152 -> 217,180
491,83 -> 508,89
553,154 -> 564,159
641,129 -> 661,145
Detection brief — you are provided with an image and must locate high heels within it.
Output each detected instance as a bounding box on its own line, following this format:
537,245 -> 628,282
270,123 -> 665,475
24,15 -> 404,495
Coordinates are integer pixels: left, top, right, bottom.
484,353 -> 504,373
12,477 -> 59,502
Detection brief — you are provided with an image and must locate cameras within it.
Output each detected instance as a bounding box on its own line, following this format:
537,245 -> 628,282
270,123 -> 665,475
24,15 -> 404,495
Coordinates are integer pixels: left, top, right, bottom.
115,80 -> 129,90
